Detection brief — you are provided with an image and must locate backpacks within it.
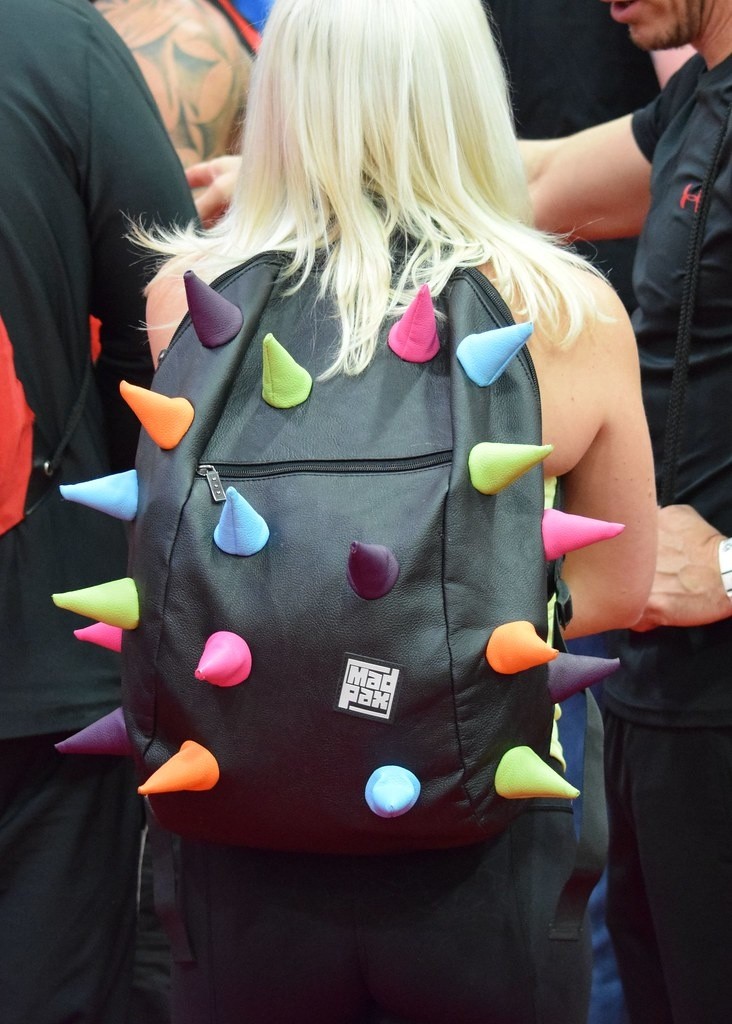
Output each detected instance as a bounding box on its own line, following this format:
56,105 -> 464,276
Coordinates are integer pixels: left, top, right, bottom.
58,233 -> 628,854
0,310 -> 103,537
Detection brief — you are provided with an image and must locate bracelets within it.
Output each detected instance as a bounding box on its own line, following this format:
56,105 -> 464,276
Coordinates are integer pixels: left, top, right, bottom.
719,538 -> 732,599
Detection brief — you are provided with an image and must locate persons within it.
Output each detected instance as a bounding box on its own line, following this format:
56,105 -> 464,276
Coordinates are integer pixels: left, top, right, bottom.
0,1 -> 732,1024
